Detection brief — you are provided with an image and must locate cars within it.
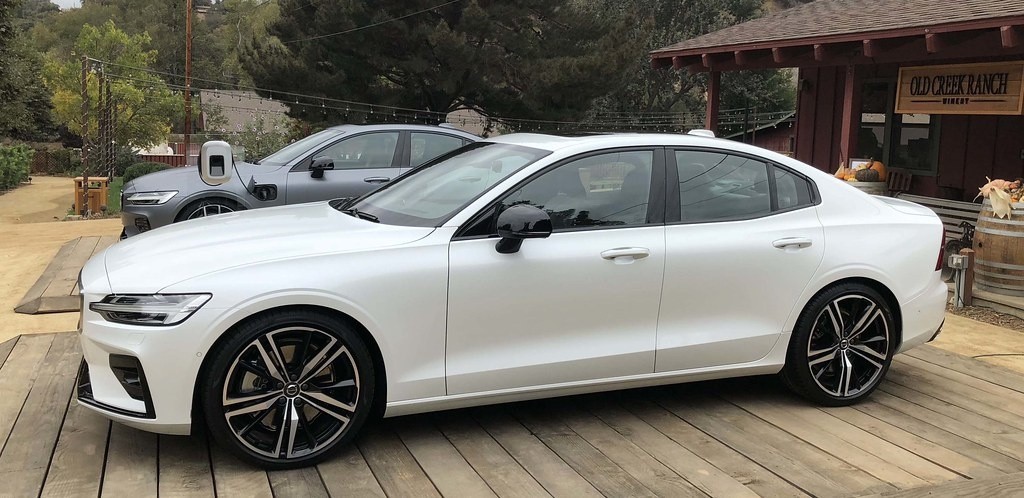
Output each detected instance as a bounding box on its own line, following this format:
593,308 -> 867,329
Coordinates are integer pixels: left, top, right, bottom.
74,129 -> 948,471
119,123 -> 591,242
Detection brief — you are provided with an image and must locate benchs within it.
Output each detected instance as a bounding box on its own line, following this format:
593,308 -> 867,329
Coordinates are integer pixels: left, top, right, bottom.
680,164 -> 790,221
893,191 -> 982,283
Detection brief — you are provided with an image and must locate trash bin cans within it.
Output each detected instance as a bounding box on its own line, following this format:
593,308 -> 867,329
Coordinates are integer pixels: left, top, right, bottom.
73,176 -> 110,215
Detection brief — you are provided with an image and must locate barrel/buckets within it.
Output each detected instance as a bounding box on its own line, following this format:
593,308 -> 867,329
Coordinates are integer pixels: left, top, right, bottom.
848,181 -> 889,196
971,198 -> 1024,296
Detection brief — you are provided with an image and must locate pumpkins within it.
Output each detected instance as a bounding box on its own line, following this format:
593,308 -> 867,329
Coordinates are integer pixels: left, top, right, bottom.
835,157 -> 886,182
979,179 -> 1024,204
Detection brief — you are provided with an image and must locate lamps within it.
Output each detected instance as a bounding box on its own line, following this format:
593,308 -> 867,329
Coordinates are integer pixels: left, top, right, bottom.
798,79 -> 814,91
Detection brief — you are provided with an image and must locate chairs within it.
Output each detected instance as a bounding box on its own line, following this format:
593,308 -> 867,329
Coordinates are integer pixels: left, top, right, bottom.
544,167 -> 590,218
620,169 -> 651,201
886,167 -> 912,198
